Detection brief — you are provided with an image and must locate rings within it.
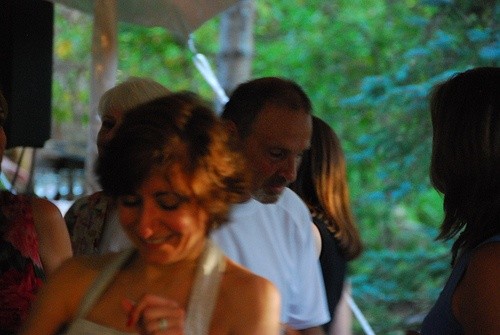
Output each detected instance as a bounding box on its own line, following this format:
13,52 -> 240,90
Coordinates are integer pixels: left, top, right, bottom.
158,317 -> 169,331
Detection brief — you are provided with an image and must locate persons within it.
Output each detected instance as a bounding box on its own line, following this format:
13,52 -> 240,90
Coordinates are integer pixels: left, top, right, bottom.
419,67 -> 500,335
17,90 -> 282,335
209,76 -> 331,335
63,77 -> 176,256
0,92 -> 75,335
287,116 -> 362,335
